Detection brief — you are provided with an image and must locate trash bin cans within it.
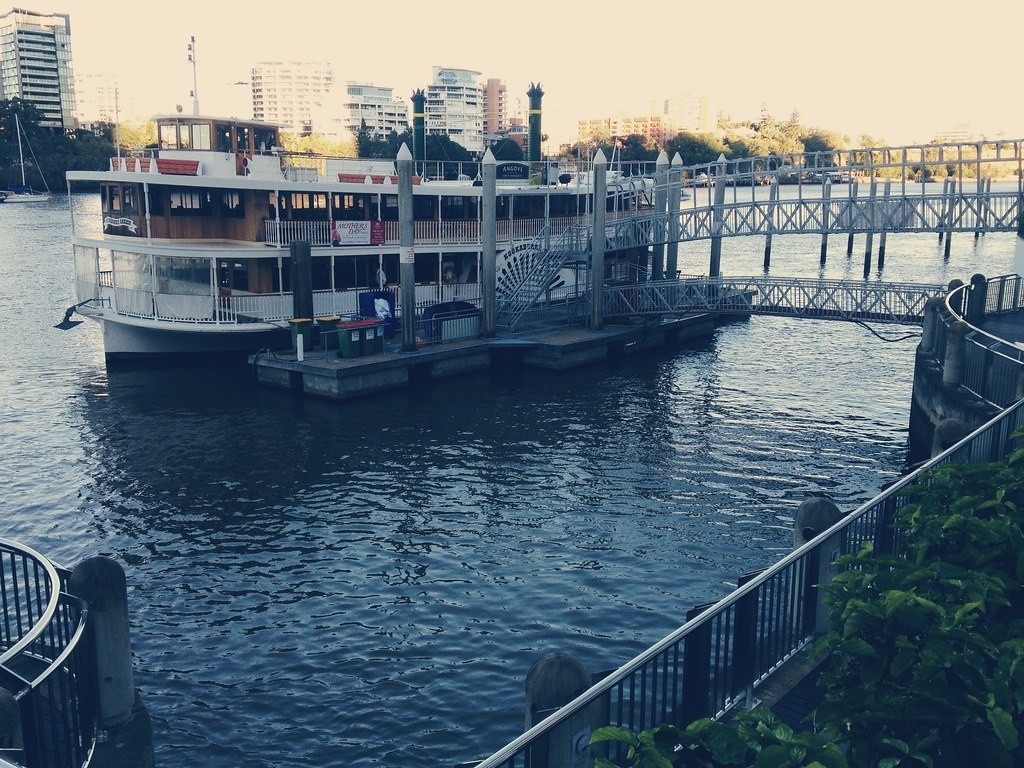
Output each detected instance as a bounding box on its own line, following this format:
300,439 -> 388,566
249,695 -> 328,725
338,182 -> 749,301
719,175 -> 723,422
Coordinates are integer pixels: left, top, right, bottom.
288,318 -> 315,353
316,316 -> 341,350
336,318 -> 386,359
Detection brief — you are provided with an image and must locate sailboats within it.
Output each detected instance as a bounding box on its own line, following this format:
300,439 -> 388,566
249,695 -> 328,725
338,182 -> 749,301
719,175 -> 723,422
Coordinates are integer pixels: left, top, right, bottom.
0,112 -> 55,203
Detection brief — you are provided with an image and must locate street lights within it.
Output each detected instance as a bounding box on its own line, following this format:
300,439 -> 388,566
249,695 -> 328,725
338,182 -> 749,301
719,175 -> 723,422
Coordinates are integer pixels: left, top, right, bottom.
186,35 -> 201,115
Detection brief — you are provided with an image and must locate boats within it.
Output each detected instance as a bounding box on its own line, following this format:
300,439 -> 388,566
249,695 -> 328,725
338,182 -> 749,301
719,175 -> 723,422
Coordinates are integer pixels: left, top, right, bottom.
571,136 -> 626,186
51,86 -> 658,366
